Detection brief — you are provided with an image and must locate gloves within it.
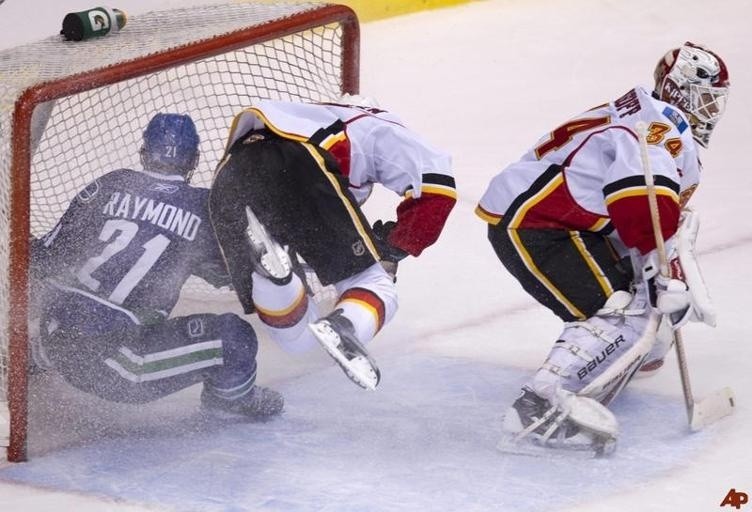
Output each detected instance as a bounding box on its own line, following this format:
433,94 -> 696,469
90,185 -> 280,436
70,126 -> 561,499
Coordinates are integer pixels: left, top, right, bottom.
373,220 -> 409,262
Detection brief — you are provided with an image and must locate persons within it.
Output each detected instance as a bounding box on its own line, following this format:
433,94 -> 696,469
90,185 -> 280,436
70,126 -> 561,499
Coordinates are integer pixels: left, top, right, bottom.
206,96 -> 457,388
475,42 -> 730,457
5,112 -> 283,421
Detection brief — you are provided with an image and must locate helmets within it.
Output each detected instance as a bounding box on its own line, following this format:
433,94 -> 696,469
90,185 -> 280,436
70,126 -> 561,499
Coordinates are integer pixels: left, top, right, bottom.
337,93 -> 377,106
139,113 -> 200,183
651,41 -> 731,149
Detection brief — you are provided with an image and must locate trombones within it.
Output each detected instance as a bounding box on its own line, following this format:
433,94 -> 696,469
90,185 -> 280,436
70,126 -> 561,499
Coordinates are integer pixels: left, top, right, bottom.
634,122 -> 735,432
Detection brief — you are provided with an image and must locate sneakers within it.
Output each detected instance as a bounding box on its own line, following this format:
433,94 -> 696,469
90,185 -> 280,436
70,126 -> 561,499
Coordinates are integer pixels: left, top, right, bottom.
317,308 -> 380,389
245,225 -> 292,286
201,385 -> 284,417
513,389 -> 616,458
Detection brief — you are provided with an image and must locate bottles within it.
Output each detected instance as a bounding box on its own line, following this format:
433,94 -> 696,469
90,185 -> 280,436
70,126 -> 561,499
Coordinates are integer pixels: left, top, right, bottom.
62,7 -> 127,41
